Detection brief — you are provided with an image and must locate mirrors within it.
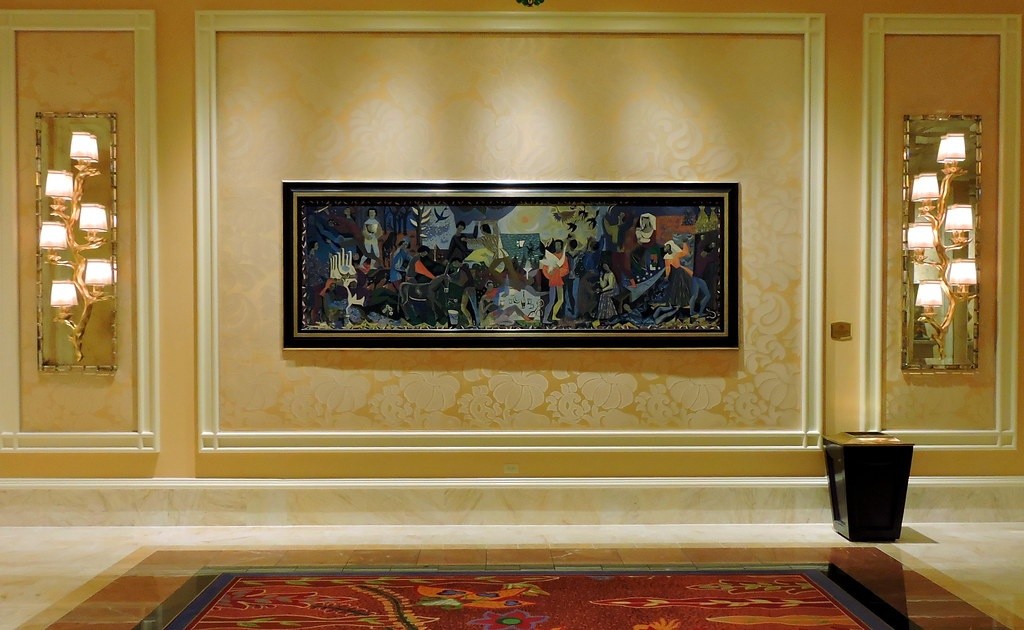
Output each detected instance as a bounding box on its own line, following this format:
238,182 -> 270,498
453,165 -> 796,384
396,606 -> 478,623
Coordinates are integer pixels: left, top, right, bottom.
902,113 -> 978,375
33,111 -> 118,377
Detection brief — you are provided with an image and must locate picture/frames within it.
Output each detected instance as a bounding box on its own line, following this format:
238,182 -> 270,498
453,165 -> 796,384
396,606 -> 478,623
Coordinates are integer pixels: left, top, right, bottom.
283,179 -> 743,350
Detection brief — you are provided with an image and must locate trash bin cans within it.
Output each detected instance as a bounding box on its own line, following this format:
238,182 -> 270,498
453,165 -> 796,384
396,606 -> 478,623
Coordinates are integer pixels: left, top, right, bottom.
819,430 -> 915,543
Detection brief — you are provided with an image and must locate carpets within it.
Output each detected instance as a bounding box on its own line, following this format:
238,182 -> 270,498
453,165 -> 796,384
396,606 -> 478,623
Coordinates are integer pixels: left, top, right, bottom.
162,571 -> 895,630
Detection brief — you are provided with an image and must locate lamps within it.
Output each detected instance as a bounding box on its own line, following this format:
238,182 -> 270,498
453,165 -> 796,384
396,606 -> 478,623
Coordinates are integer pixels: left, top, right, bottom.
40,130 -> 112,364
907,133 -> 978,361
